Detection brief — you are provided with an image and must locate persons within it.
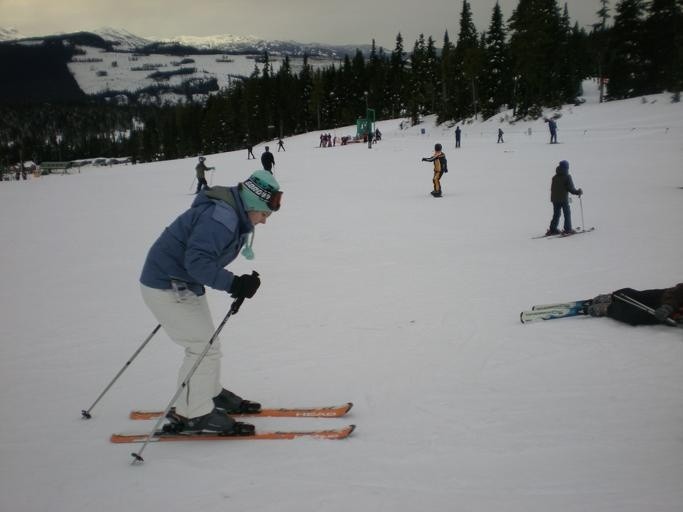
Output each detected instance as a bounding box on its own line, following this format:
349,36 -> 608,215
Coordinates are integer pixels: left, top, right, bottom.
455,126 -> 461,146
363,128 -> 382,149
546,160 -> 582,237
319,131 -> 349,148
139,170 -> 283,434
261,146 -> 276,176
421,144 -> 447,197
276,138 -> 286,152
586,283 -> 683,327
245,142 -> 256,160
194,156 -> 215,194
549,118 -> 559,144
496,128 -> 505,143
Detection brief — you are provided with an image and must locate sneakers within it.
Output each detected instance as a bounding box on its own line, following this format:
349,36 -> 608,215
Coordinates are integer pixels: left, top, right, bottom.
175,388 -> 243,434
587,294 -> 612,317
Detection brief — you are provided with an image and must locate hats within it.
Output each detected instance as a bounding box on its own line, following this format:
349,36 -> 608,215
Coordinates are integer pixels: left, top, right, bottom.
238,170 -> 282,212
560,160 -> 568,169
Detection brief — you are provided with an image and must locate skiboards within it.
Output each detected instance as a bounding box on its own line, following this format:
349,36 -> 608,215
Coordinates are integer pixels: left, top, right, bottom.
111,403 -> 355,443
532,227 -> 594,239
520,300 -> 597,323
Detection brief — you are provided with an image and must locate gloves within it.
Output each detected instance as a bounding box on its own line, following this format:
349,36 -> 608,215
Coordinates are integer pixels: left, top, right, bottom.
229,275 -> 260,298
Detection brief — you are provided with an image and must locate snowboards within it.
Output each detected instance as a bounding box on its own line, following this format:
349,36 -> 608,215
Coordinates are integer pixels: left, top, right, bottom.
430,191 -> 443,199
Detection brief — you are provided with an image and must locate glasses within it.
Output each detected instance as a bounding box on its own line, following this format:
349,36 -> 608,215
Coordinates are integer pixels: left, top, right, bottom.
242,178 -> 283,211
